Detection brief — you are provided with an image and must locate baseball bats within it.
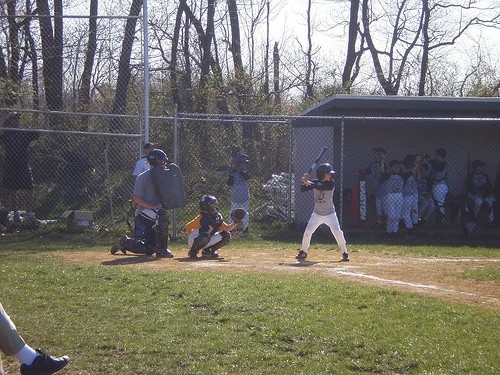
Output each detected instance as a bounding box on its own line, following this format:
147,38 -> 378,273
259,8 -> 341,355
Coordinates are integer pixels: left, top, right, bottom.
300,146 -> 328,184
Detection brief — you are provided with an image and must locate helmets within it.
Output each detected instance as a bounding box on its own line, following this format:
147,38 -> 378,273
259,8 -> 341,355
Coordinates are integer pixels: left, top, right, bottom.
200,194 -> 218,217
147,149 -> 168,166
237,154 -> 250,164
316,163 -> 335,178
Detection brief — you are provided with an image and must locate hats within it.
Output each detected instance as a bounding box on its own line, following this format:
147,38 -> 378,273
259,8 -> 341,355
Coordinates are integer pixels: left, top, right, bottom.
472,160 -> 487,167
371,147 -> 385,154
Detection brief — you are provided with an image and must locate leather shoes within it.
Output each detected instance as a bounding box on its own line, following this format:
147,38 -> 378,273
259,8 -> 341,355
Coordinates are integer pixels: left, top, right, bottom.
20,348 -> 69,375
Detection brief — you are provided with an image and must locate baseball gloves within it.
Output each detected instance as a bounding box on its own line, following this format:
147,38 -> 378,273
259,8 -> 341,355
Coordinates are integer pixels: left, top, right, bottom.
231,209 -> 245,223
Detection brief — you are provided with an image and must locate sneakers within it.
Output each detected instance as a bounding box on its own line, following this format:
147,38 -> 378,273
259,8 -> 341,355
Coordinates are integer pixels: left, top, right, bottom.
202,250 -> 219,259
295,251 -> 307,260
156,249 -> 173,258
343,253 -> 349,262
110,235 -> 128,254
188,251 -> 200,260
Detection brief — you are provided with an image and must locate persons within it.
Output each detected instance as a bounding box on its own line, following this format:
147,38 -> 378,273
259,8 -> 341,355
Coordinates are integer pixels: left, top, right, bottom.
133,142 -> 158,179
111,149 -> 174,257
0,302 -> 70,374
186,195 -> 242,259
227,154 -> 251,234
463,159 -> 498,228
369,146 -> 449,239
295,163 -> 349,262
0,108 -> 53,229
